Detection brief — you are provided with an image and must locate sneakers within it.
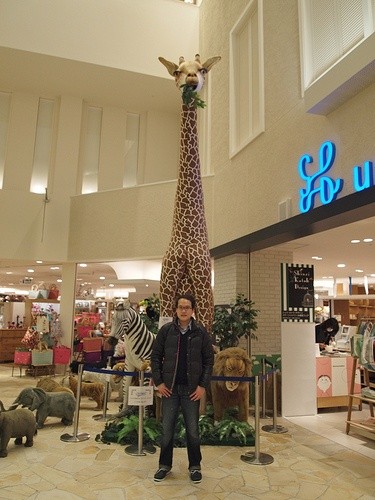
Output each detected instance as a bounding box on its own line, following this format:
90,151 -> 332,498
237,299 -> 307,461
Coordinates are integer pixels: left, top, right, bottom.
153,468 -> 172,482
189,470 -> 203,483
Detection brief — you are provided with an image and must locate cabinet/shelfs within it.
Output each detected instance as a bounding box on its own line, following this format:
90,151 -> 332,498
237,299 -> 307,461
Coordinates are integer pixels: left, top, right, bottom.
315,284 -> 375,328
74,300 -> 109,325
344,356 -> 375,441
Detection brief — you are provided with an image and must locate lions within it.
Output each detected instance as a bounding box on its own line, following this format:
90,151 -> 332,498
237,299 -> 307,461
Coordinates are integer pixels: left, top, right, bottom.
200,346 -> 252,428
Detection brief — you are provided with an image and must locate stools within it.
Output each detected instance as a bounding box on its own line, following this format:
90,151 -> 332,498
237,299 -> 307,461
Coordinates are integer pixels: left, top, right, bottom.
30,366 -> 52,378
12,364 -> 32,377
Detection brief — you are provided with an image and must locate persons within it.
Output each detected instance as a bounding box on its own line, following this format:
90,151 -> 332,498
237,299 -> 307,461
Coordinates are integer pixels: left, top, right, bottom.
151,294 -> 214,483
315,318 -> 339,351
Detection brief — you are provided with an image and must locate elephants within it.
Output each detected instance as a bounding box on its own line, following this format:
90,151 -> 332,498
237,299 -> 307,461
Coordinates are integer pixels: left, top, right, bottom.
0,387 -> 77,458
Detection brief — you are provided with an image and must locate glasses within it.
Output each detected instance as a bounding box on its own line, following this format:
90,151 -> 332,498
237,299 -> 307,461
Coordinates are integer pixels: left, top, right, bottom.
176,305 -> 192,311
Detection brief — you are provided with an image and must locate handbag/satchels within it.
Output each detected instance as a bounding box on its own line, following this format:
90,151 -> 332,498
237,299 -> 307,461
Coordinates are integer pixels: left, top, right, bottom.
21,327 -> 37,350
28,283 -> 59,299
77,317 -> 102,363
14,342 -> 70,366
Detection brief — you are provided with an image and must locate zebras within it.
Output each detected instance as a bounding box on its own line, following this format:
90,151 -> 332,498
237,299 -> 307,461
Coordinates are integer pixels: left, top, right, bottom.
107,297 -> 158,417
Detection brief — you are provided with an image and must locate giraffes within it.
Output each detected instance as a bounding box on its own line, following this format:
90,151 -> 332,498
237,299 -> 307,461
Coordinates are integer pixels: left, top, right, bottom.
159,53 -> 221,332
